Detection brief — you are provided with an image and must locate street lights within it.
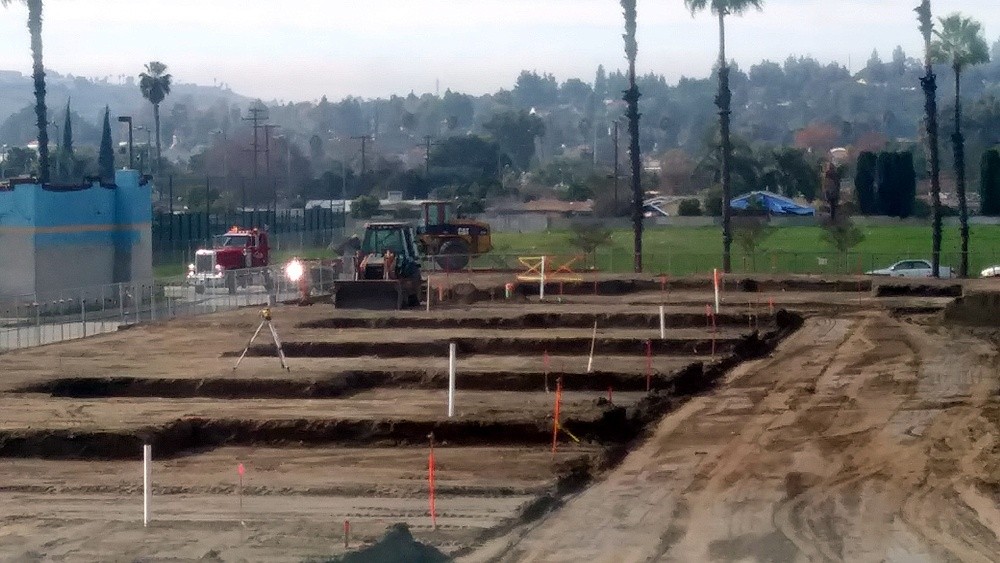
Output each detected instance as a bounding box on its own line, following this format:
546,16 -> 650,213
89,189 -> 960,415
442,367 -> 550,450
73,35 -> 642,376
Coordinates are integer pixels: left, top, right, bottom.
117,116 -> 133,168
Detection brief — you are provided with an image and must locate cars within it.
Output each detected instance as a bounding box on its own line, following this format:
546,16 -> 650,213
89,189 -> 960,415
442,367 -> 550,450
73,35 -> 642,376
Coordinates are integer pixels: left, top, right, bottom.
187,226 -> 276,294
982,266 -> 1000,278
864,259 -> 955,280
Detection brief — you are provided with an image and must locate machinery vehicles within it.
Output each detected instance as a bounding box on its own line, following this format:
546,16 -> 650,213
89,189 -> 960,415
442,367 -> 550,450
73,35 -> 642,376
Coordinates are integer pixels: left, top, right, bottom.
334,221 -> 422,310
331,200 -> 493,270
